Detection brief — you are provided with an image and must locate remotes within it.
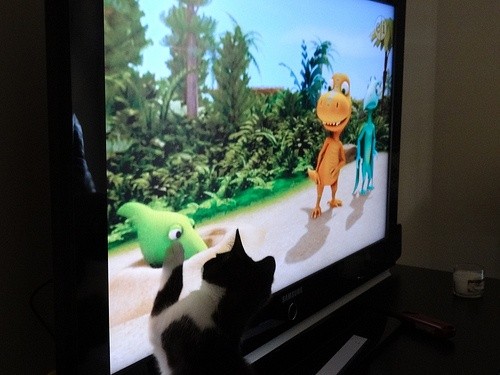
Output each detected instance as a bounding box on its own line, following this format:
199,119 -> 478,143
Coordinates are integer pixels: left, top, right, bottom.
400,311 -> 456,337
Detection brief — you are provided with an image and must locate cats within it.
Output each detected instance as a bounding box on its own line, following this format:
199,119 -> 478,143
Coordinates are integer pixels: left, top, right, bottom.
147,228 -> 276,375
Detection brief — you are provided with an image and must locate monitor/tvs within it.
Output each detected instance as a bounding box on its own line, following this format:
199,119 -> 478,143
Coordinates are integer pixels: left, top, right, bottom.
43,0 -> 403,375
